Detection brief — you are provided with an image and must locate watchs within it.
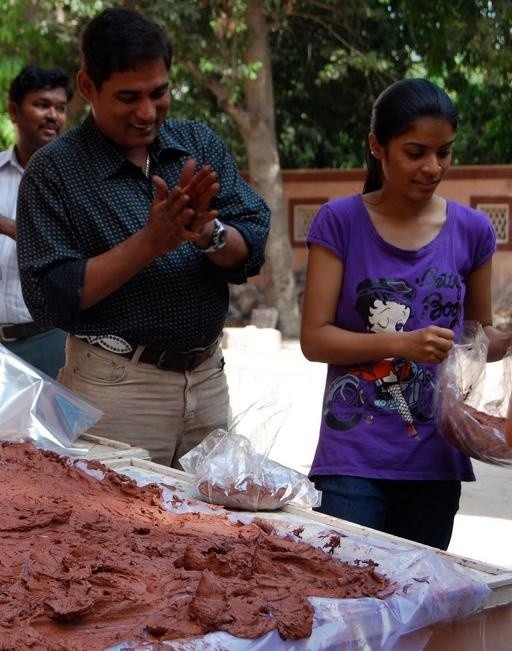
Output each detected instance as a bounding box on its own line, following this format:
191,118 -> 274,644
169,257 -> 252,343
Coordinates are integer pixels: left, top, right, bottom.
199,217 -> 228,254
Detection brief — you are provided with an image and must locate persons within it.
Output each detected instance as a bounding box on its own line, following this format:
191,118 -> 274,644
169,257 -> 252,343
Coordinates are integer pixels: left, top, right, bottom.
14,9 -> 269,470
296,79 -> 512,554
1,62 -> 77,382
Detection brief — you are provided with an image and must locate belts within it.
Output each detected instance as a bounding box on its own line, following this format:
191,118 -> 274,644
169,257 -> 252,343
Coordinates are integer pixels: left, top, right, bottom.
0,323 -> 59,344
76,331 -> 221,374
